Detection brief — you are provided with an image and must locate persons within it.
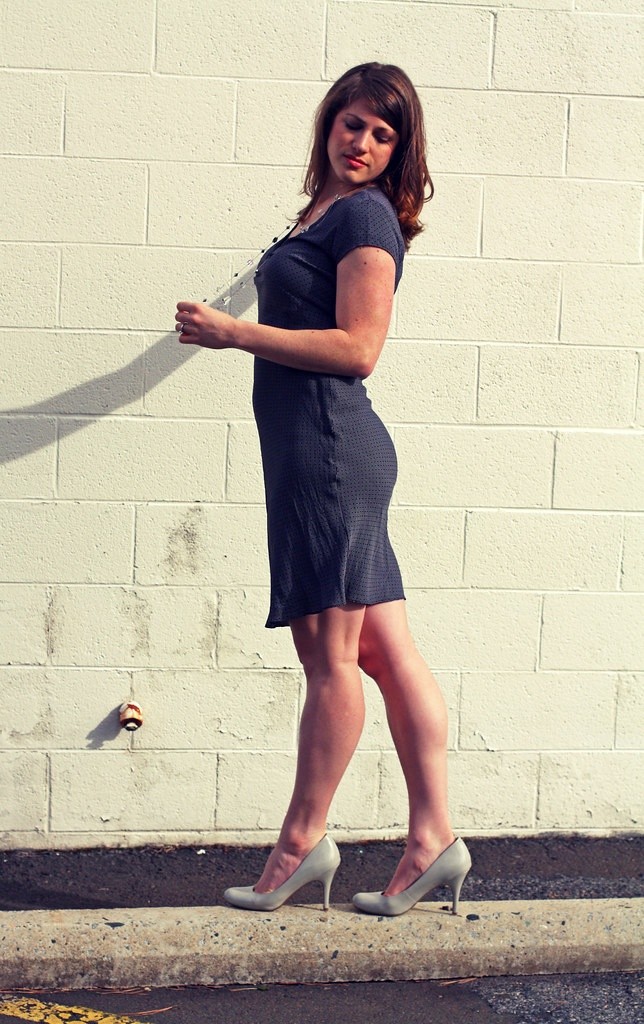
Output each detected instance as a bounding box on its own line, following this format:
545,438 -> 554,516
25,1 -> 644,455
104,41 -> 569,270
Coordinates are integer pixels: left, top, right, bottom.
174,62 -> 473,915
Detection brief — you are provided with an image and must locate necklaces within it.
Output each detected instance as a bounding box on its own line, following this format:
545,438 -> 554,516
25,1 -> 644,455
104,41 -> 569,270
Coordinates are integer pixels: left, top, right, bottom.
203,191 -> 339,309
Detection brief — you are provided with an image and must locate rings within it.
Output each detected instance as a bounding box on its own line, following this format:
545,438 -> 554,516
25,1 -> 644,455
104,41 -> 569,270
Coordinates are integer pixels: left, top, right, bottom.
179,322 -> 188,335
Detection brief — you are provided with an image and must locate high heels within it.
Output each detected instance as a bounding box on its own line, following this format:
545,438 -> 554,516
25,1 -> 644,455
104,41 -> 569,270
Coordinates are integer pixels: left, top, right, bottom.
223,833 -> 341,911
352,836 -> 472,916
